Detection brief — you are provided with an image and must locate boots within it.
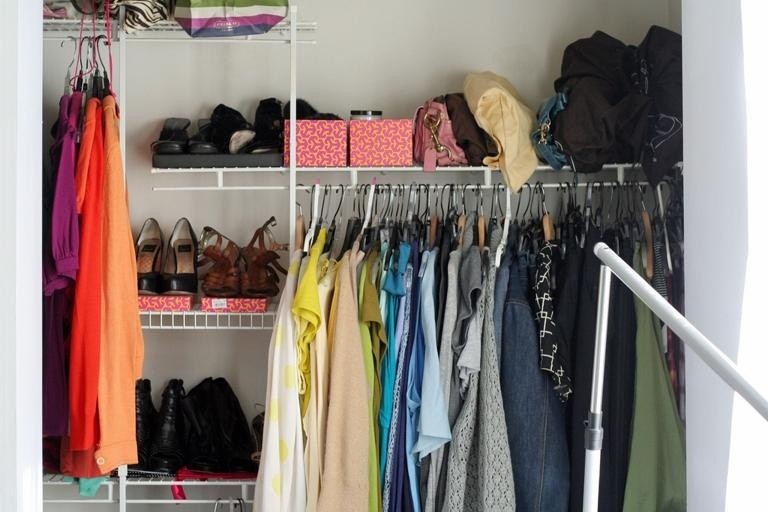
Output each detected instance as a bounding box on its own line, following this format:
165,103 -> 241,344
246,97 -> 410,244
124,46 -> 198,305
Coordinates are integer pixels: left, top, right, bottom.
135,378 -> 253,474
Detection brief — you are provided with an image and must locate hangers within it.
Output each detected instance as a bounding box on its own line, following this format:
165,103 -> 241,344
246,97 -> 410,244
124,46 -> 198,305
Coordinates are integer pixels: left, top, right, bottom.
59,29 -> 113,100
297,182 -> 683,278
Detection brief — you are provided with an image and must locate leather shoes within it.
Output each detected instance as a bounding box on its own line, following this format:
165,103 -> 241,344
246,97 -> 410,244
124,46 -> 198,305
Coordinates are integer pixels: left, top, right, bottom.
151,96 -> 284,154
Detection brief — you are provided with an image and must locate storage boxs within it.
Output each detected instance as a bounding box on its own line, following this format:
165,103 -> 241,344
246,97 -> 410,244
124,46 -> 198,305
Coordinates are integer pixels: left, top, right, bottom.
283,117 -> 413,167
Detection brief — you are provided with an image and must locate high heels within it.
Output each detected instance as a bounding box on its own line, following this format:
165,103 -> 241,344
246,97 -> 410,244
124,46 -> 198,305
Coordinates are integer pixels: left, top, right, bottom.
134,217 -> 280,297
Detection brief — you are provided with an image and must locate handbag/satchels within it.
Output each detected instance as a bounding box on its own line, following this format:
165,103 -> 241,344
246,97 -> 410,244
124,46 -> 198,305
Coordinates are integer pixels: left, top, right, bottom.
413,71 -> 538,193
